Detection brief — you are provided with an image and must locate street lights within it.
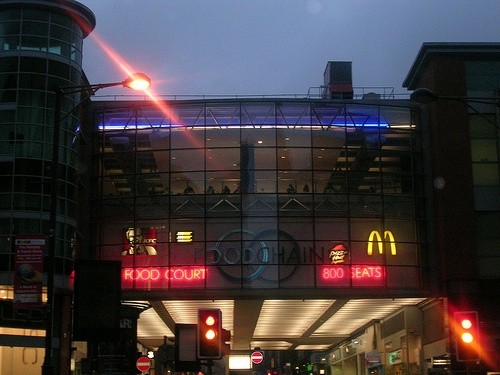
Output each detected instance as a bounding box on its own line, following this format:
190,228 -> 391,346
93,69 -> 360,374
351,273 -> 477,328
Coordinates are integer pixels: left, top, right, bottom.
41,73 -> 151,374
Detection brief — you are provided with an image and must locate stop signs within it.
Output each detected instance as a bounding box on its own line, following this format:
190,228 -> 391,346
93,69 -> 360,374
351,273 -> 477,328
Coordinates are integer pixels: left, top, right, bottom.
136,357 -> 152,372
252,350 -> 263,364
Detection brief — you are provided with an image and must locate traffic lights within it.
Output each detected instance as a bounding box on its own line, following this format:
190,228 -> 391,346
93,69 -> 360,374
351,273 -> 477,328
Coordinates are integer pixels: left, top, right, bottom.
197,309 -> 231,363
454,312 -> 481,364
